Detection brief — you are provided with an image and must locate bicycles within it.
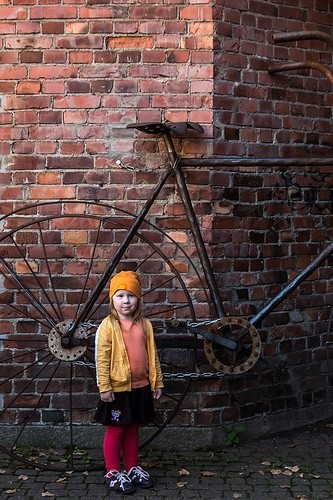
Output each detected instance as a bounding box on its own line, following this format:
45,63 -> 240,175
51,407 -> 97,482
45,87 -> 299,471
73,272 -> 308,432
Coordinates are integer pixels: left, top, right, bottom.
0,30 -> 333,472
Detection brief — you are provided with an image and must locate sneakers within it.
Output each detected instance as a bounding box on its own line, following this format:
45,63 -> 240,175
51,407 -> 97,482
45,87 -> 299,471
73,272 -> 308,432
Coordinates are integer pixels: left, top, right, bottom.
104,474 -> 136,494
124,467 -> 155,489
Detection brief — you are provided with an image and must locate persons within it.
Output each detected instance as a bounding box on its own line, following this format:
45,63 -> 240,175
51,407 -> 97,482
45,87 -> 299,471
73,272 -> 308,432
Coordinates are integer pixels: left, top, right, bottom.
94,271 -> 165,494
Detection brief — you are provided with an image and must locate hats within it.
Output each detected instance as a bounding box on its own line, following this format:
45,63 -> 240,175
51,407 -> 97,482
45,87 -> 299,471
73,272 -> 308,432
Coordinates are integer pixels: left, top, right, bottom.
109,271 -> 142,301
